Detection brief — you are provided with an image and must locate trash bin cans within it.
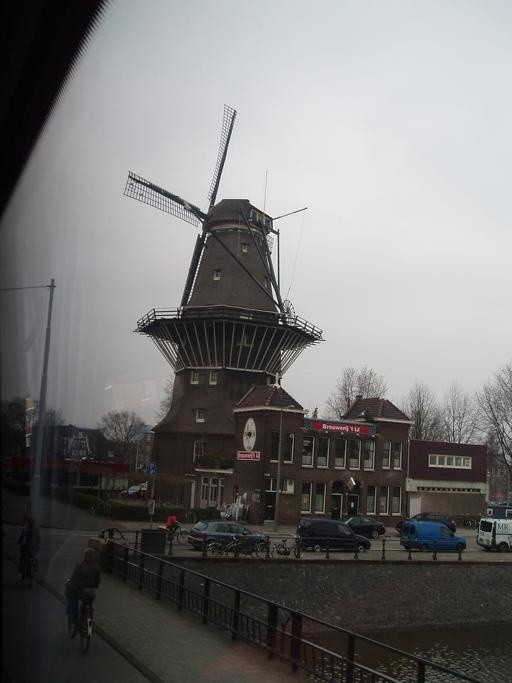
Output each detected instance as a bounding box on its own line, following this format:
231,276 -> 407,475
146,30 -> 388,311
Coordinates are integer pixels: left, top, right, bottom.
141,529 -> 165,554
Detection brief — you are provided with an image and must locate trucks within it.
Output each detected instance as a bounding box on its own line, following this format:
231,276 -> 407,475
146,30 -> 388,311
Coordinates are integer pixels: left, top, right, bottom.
477,518 -> 512,553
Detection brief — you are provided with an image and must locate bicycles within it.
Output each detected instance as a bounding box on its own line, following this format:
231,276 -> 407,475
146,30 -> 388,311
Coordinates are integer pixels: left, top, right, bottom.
204,534 -> 306,558
18,540 -> 32,588
158,521 -> 189,545
64,579 -> 93,655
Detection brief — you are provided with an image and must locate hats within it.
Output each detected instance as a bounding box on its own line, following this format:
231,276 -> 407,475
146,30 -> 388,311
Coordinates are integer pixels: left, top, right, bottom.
85,548 -> 95,562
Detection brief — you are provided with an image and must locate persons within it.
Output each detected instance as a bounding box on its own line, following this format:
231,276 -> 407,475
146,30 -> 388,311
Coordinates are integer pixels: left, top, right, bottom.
15,515 -> 40,577
167,510 -> 181,540
65,547 -> 102,640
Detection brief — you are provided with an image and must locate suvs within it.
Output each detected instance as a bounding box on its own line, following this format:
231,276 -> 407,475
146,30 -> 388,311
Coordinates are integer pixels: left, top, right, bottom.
296,512 -> 467,553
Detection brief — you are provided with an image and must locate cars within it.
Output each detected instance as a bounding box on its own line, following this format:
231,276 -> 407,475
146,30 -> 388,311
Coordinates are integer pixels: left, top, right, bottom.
121,482 -> 147,500
188,521 -> 268,551
